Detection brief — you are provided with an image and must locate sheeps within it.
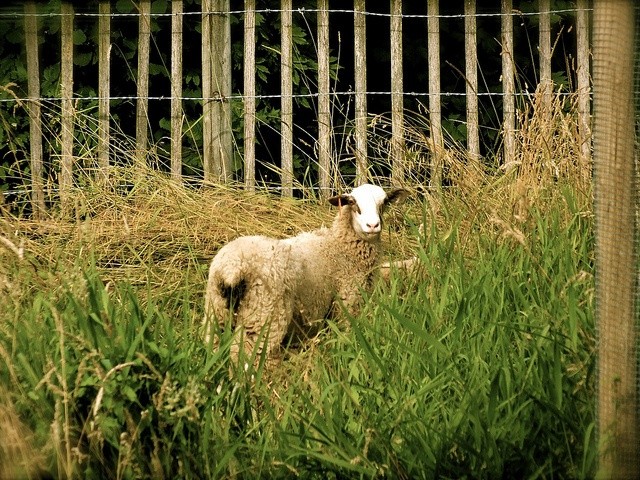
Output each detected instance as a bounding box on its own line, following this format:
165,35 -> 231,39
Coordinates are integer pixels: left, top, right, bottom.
203,183 -> 413,381
380,259 -> 414,285
